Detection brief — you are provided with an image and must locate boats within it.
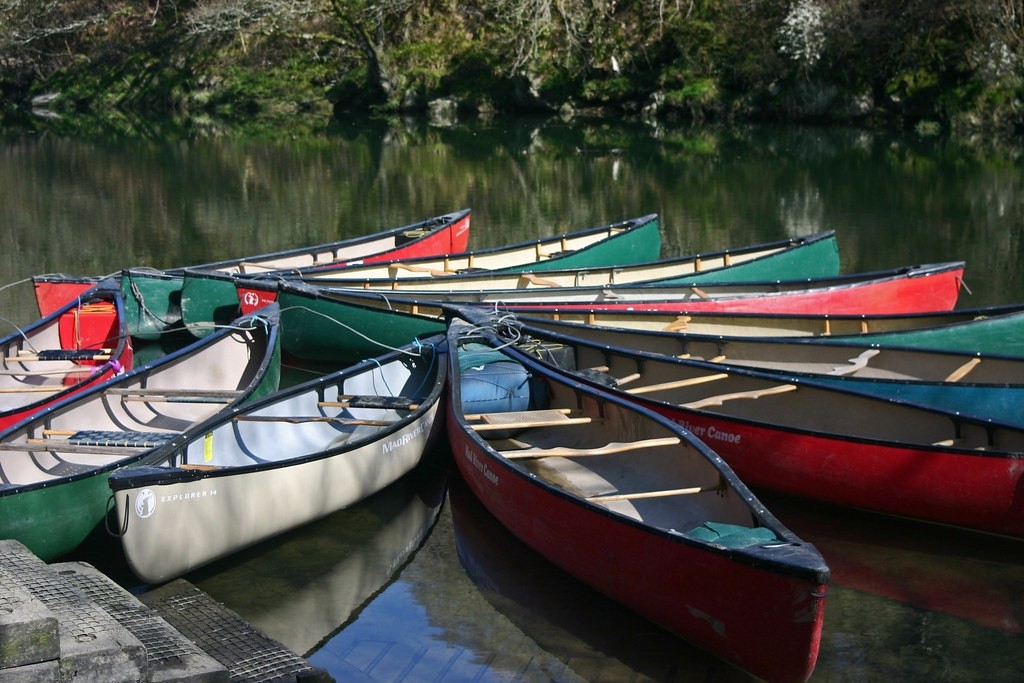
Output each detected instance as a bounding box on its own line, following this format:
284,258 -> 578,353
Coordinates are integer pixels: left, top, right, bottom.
0,208 -> 1024,683
